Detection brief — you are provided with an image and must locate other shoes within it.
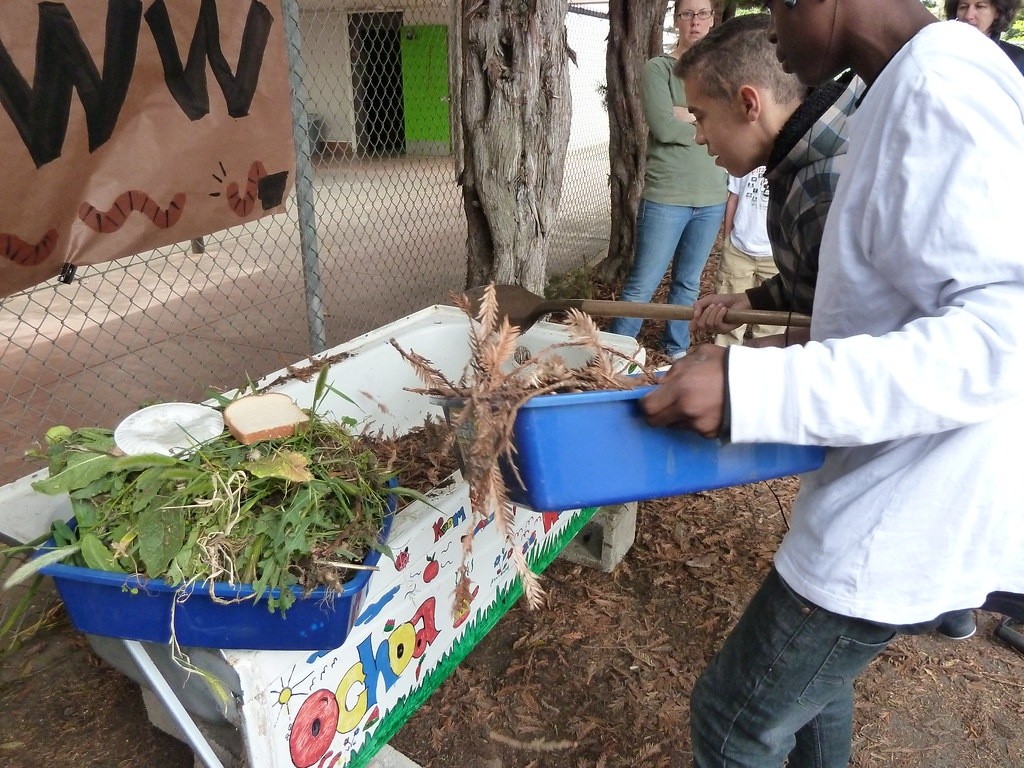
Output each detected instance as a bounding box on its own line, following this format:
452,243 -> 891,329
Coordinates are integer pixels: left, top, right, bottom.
670,351 -> 686,361
934,614 -> 978,640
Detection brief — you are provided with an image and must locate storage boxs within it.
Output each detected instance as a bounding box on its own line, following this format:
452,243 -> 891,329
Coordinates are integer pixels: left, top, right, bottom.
32,479 -> 403,654
430,378 -> 825,512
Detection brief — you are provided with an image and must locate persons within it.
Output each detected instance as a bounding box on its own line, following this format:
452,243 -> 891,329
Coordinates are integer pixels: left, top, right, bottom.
607,0 -> 1024,768
673,14 -> 977,640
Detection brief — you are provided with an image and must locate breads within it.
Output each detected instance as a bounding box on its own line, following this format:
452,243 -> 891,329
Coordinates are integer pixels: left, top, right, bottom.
222,393 -> 311,445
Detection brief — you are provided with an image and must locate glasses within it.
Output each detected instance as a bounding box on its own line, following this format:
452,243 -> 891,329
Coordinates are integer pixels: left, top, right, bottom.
674,9 -> 714,20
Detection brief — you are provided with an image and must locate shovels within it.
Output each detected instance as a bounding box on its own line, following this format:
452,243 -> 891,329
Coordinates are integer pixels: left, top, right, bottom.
461,281 -> 811,339
